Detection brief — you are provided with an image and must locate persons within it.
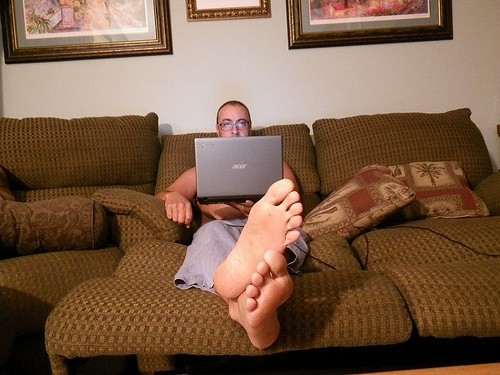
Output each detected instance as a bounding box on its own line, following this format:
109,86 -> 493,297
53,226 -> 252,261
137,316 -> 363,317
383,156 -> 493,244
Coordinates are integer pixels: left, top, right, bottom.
155,98 -> 311,350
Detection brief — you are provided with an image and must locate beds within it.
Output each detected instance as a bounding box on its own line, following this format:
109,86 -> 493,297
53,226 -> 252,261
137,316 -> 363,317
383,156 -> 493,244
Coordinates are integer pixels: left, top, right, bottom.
0,108 -> 500,375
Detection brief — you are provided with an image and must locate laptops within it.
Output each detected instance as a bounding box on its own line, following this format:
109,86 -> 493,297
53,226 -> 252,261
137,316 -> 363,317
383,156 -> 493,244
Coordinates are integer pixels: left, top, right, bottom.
197,136 -> 283,202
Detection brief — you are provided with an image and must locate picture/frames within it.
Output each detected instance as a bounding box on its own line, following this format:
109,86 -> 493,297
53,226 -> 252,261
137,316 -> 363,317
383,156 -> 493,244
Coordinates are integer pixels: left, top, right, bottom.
186,0 -> 271,22
286,0 -> 453,49
0,0 -> 173,64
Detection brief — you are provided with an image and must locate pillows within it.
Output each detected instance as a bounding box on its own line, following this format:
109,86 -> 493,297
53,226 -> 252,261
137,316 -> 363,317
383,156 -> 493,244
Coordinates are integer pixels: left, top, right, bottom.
300,165 -> 417,240
0,196 -> 107,254
388,161 -> 490,217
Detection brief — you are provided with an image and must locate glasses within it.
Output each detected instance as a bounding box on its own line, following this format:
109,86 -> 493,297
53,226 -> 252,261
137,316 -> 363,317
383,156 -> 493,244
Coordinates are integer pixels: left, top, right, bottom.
218,121 -> 250,130
